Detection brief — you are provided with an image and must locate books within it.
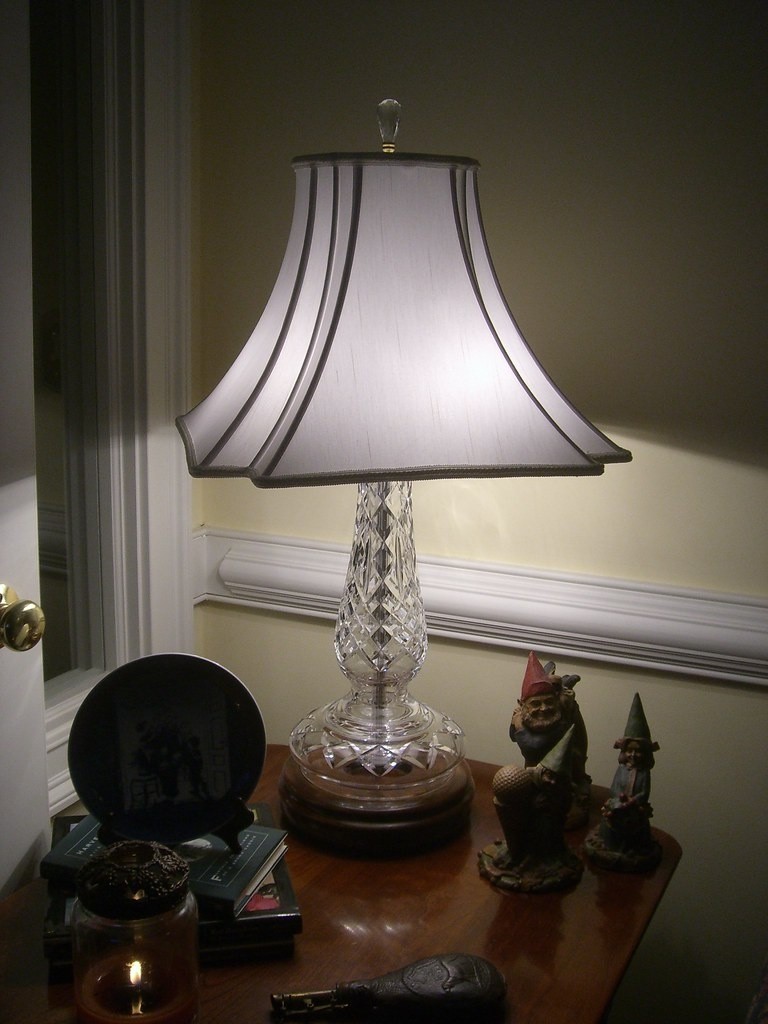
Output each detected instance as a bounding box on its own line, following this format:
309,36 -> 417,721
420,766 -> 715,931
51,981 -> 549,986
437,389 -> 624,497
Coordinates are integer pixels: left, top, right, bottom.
40,802 -> 301,983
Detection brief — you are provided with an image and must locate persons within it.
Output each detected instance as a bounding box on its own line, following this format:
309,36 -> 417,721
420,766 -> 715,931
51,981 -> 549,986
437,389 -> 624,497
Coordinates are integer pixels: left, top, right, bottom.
507,692 -> 658,866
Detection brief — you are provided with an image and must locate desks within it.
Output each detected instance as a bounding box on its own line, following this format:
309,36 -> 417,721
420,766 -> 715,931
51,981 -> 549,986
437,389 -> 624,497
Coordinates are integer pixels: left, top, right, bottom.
0,744 -> 685,1024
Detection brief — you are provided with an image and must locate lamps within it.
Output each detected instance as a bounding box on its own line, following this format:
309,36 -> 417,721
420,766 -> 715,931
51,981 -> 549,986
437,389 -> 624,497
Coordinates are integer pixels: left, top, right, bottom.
173,96 -> 634,865
